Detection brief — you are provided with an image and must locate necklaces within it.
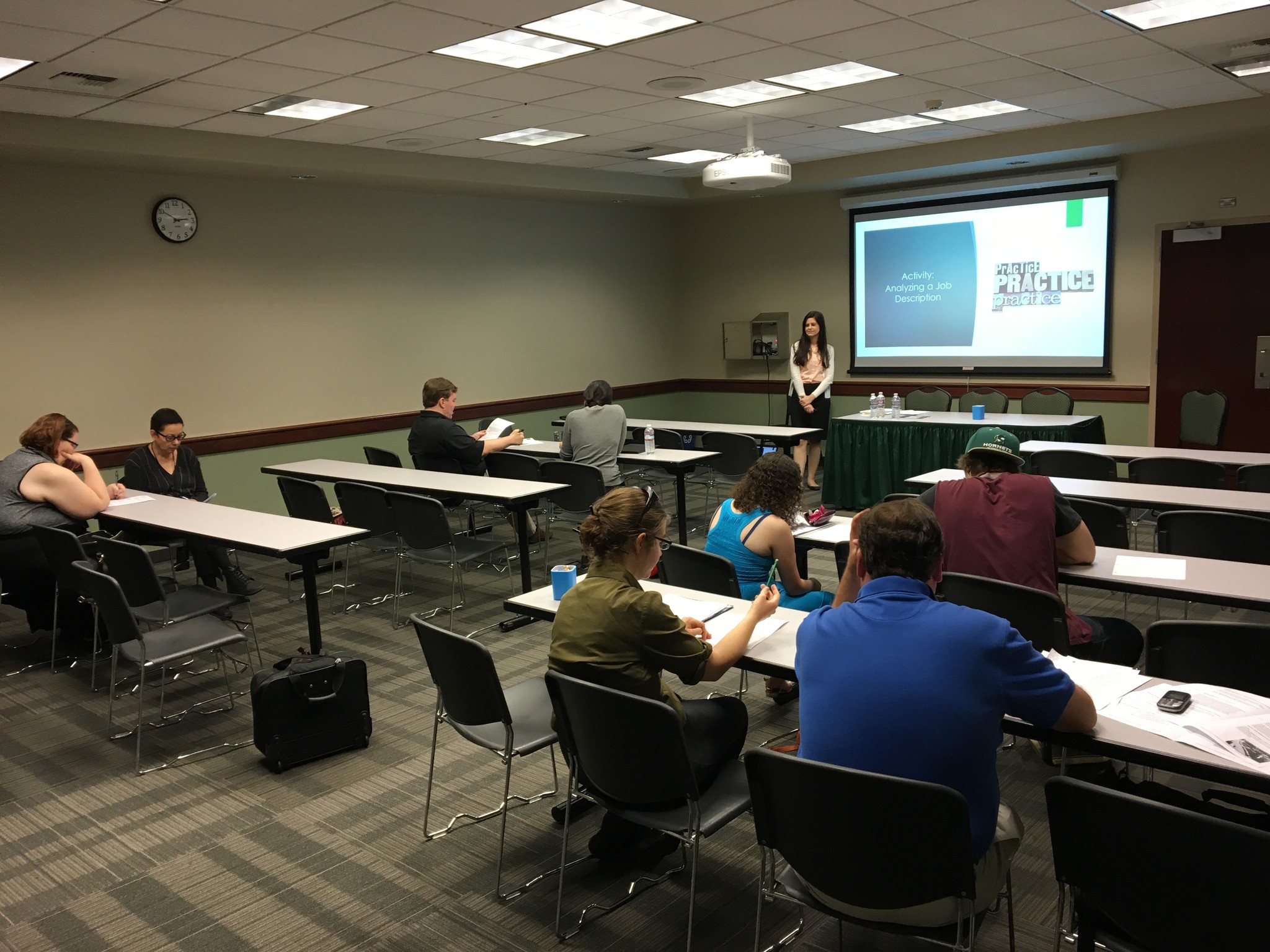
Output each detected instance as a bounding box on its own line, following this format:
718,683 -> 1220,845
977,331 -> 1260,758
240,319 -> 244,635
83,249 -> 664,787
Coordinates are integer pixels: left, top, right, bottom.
151,441 -> 176,467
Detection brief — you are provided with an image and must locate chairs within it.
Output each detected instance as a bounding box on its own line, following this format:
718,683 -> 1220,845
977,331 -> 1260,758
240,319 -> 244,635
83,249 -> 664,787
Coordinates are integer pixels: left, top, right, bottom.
1063,497 -> 1133,620
1177,387 -> 1228,450
1145,619 -> 1270,698
363,446 -> 402,468
1030,451 -> 1117,481
542,671 -> 804,952
412,454 -> 495,539
1044,776 -> 1270,952
540,461 -> 607,585
938,572 -> 1068,655
656,543 -> 748,700
334,482 -> 414,616
118,477 -> 239,591
959,387 -> 1009,413
632,427 -> 715,519
1021,387 -> 1074,415
277,475 -> 362,616
1128,457 -> 1227,552
904,386 -> 952,412
1152,510 -> 1270,620
408,614 -> 593,902
92,535 -> 264,719
743,748 -> 1014,952
486,452 -> 542,561
701,431 -> 757,538
72,561 -> 254,774
27,522 -> 196,692
384,491 -> 518,638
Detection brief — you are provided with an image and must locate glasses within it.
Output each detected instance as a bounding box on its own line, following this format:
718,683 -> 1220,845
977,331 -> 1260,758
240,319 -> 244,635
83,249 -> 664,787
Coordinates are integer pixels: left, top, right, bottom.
637,485 -> 659,528
62,437 -> 78,449
650,534 -> 672,550
156,431 -> 186,442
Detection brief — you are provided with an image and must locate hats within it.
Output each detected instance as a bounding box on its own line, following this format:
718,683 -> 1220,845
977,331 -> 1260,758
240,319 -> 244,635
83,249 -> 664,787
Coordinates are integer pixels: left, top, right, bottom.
965,427 -> 1025,467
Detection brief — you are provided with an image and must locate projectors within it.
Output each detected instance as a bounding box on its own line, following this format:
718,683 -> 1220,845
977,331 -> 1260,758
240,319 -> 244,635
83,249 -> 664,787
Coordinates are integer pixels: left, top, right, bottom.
702,157 -> 792,192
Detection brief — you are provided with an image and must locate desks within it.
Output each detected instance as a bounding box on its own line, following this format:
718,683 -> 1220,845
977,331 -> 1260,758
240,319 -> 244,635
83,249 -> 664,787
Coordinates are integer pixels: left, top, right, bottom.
100,408 -> 1270,795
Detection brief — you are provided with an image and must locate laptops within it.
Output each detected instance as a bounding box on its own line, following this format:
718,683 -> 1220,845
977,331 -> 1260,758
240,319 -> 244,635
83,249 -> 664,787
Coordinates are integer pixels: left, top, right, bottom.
756,444 -> 778,456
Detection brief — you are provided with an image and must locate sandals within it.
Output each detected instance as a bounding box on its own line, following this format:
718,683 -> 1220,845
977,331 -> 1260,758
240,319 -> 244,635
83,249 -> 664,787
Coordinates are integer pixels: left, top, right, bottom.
766,682 -> 792,697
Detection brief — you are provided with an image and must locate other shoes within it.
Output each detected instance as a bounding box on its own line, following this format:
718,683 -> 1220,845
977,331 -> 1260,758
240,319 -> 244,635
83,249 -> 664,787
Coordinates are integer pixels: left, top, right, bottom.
808,484 -> 820,490
590,831 -> 679,869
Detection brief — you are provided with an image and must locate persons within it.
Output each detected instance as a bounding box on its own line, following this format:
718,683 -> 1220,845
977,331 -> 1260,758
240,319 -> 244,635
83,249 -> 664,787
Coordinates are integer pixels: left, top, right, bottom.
122,408 -> 265,620
0,412 -> 125,660
560,380 -> 628,496
706,451 -> 836,700
916,427 -> 1143,665
549,486 -> 780,872
407,377 -> 555,545
783,497 -> 1097,929
790,311 -> 835,491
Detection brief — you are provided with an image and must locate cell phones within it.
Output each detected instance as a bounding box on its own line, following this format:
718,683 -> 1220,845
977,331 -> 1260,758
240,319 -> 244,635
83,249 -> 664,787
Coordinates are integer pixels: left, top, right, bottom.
1157,690 -> 1191,712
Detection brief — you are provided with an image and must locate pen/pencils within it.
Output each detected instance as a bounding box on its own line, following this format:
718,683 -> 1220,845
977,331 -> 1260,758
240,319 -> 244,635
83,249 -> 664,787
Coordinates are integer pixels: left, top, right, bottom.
116,470 -> 118,496
766,559 -> 779,587
509,429 -> 524,434
180,496 -> 189,499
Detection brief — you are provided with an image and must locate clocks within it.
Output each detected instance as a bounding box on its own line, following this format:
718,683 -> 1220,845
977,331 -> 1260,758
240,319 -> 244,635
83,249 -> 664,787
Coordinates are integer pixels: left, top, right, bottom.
151,197 -> 198,243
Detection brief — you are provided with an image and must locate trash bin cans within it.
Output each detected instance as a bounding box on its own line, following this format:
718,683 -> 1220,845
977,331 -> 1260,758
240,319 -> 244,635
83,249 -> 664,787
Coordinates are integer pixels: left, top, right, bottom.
972,405 -> 985,420
551,565 -> 576,600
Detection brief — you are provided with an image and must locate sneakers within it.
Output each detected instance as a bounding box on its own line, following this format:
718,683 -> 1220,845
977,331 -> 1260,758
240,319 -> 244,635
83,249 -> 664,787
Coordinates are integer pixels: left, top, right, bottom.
213,608 -> 232,619
224,565 -> 267,595
528,527 -> 553,543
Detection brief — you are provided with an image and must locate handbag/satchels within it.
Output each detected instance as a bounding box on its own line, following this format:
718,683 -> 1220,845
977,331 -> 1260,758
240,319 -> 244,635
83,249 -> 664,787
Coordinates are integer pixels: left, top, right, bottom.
251,647 -> 372,771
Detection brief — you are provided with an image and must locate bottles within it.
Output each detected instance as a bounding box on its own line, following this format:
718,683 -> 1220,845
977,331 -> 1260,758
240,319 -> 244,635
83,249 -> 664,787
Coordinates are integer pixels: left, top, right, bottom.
877,392 -> 885,416
644,424 -> 655,454
869,393 -> 878,417
891,393 -> 900,419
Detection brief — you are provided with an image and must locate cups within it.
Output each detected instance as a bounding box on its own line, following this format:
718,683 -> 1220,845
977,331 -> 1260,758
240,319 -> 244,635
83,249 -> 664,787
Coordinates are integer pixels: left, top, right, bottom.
972,405 -> 986,420
551,565 -> 576,600
